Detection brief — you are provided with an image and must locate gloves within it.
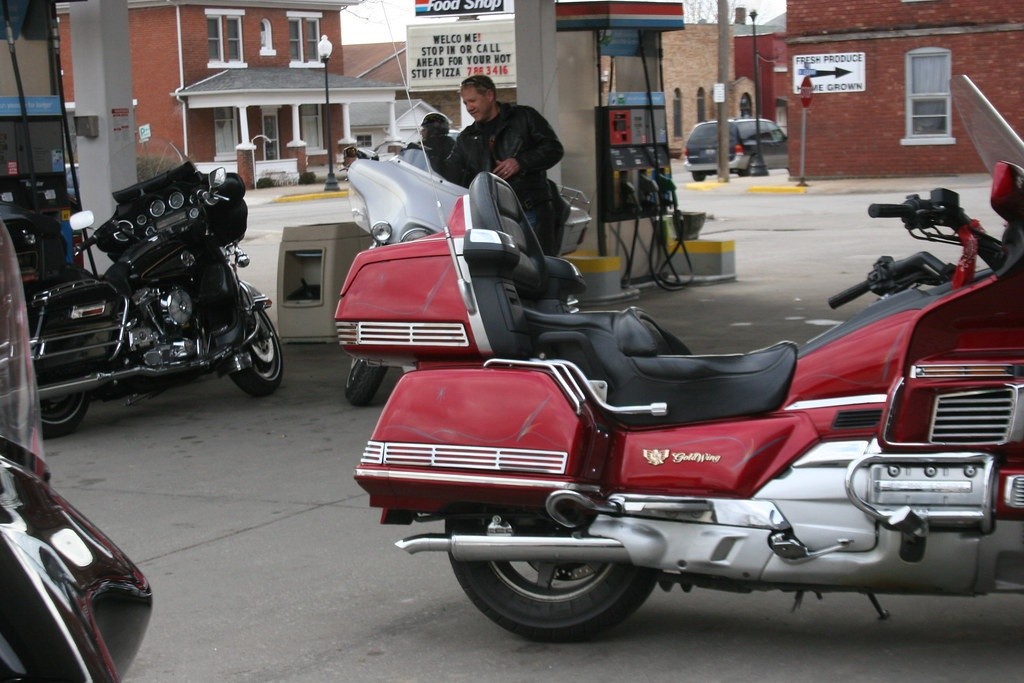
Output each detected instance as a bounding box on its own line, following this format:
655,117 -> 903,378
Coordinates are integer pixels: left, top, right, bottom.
407,142 -> 420,149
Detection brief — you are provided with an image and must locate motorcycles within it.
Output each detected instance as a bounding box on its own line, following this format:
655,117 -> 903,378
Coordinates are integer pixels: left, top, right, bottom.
0,220 -> 156,683
335,131 -> 592,406
333,75 -> 1022,645
1,135 -> 284,442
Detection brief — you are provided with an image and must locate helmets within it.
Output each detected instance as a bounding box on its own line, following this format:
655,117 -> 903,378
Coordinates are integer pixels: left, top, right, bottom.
421,113 -> 449,137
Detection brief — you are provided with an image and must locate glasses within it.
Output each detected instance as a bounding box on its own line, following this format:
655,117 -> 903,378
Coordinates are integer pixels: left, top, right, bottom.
462,78 -> 492,89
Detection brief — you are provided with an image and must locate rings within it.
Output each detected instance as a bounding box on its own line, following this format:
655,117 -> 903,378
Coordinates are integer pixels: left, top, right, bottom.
505,171 -> 507,174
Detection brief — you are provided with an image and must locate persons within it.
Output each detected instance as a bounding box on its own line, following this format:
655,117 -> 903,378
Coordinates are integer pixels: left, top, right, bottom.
401,74 -> 571,256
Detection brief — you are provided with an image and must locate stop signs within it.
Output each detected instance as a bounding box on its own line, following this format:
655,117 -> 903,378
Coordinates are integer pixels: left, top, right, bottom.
800,78 -> 814,109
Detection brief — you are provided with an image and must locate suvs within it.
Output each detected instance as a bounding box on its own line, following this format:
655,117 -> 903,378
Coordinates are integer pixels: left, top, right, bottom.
683,117 -> 787,182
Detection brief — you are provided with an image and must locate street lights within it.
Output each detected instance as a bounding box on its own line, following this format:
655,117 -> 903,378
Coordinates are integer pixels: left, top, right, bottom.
314,33 -> 341,193
744,7 -> 768,176
